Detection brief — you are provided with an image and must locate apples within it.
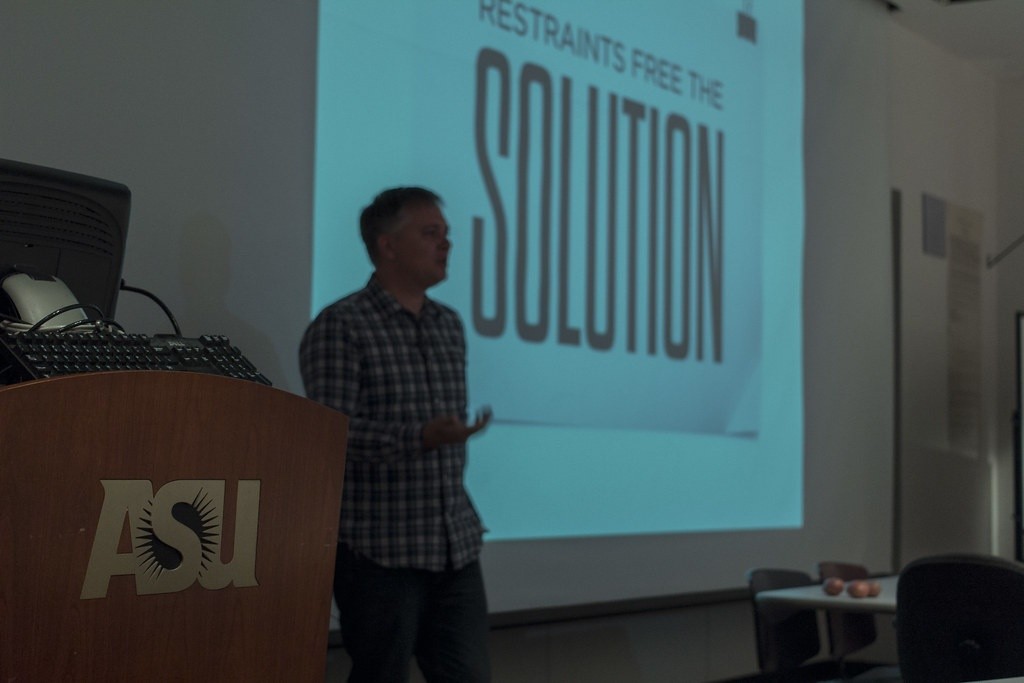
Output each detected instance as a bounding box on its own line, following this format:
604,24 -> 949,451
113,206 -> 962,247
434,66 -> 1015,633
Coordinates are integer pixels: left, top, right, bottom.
822,578 -> 881,598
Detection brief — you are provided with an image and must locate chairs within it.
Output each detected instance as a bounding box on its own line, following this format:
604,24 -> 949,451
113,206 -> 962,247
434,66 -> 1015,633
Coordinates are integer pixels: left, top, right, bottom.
897,554 -> 1024,683
748,560 -> 898,683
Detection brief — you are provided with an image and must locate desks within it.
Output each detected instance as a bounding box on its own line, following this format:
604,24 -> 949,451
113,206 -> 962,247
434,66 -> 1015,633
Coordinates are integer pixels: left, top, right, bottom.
754,573 -> 900,663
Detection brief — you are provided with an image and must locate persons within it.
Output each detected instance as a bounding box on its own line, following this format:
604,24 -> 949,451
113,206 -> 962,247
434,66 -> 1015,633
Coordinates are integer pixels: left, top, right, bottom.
299,186 -> 491,683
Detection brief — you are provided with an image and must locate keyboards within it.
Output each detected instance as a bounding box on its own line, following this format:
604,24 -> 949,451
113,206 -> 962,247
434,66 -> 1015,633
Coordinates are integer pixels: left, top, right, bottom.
0,329 -> 275,384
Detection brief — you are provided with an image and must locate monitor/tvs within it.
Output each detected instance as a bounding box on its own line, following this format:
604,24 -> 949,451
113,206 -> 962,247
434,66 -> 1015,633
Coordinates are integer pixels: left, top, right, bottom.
0,158 -> 133,332
